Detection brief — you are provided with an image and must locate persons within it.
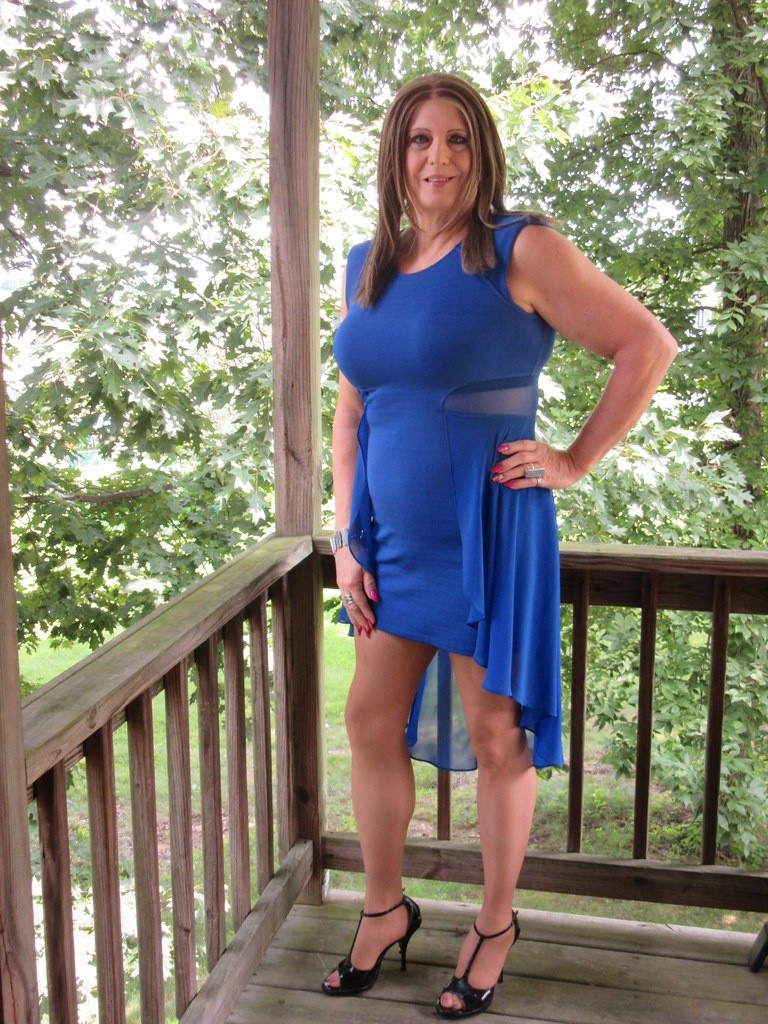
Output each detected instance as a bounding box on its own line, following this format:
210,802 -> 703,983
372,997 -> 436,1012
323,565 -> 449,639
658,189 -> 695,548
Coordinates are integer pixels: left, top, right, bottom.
321,69 -> 679,1021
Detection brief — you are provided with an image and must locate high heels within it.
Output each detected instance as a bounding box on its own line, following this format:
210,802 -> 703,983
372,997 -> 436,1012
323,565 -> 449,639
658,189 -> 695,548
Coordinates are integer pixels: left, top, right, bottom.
435,908 -> 522,1019
322,887 -> 423,996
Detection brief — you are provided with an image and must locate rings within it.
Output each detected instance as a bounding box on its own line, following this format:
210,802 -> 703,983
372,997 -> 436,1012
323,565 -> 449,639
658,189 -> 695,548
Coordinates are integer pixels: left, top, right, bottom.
343,594 -> 354,605
524,461 -> 546,489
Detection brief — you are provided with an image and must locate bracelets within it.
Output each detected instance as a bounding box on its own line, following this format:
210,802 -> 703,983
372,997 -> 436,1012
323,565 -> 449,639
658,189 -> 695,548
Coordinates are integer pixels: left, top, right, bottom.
329,528 -> 370,554
